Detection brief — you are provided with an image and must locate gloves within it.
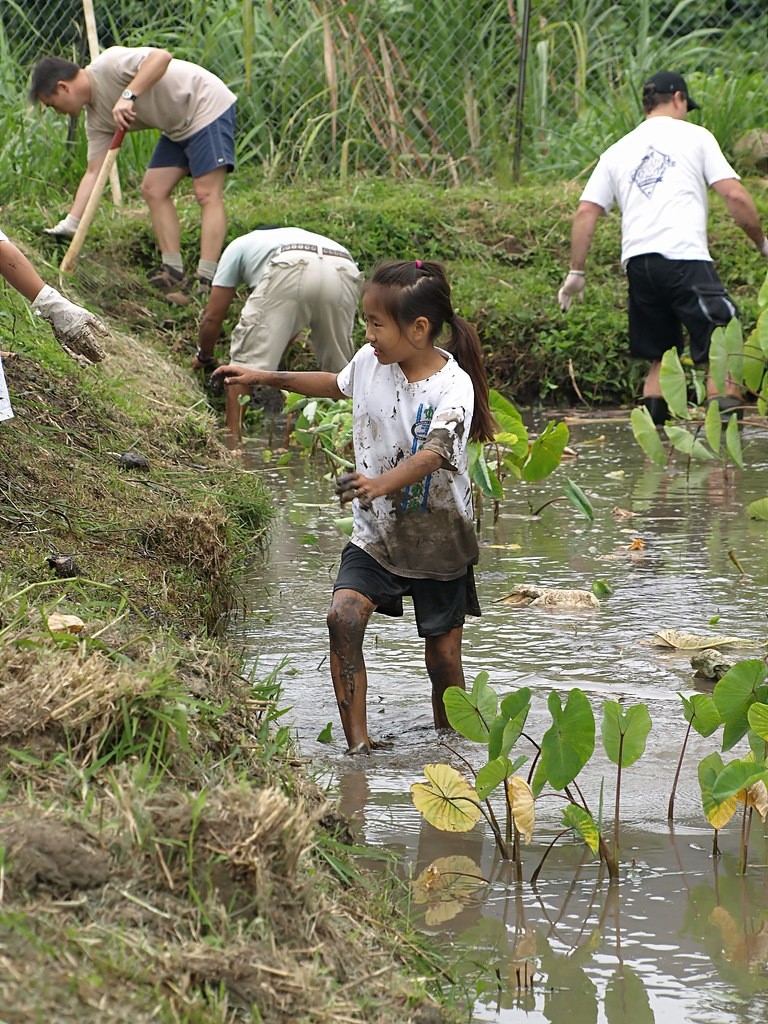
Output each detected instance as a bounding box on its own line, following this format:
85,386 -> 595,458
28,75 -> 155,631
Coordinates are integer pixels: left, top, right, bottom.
30,285 -> 108,364
558,270 -> 585,313
41,214 -> 81,241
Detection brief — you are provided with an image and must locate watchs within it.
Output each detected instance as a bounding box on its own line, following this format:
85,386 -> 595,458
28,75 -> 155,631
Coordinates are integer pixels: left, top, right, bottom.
121,88 -> 137,102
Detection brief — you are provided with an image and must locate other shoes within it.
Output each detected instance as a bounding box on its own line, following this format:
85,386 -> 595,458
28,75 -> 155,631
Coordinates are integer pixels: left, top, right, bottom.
147,264 -> 183,292
165,273 -> 213,308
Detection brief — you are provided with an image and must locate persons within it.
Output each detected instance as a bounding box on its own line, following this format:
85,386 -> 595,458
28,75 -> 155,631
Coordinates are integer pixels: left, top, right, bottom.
190,225 -> 364,450
28,43 -> 240,305
207,258 -> 502,755
557,69 -> 768,435
0,223 -> 108,424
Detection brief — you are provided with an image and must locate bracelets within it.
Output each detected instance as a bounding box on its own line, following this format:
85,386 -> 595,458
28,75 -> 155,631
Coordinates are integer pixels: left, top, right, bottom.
196,350 -> 212,364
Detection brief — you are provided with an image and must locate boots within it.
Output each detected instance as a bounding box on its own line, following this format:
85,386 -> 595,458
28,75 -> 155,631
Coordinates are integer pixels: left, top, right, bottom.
704,394 -> 744,431
634,397 -> 669,428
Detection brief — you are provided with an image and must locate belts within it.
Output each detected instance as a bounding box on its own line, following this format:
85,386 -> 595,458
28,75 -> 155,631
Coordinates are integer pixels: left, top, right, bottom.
266,243 -> 352,262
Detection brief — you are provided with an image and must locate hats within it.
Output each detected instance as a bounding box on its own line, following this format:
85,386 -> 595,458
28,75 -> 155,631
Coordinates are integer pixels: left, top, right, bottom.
643,69 -> 701,113
